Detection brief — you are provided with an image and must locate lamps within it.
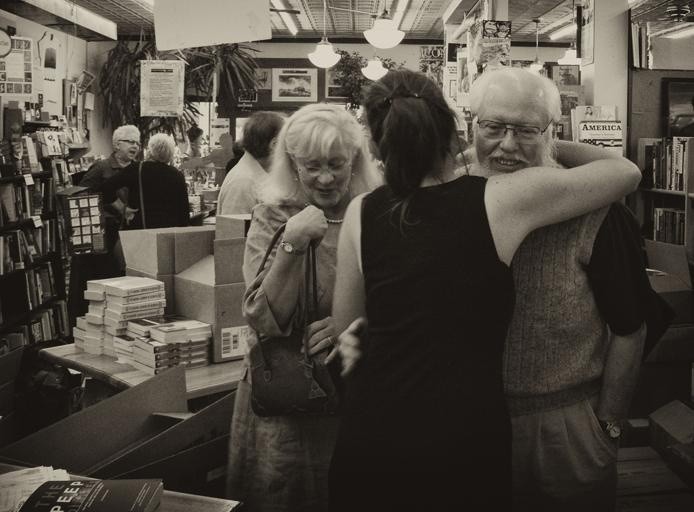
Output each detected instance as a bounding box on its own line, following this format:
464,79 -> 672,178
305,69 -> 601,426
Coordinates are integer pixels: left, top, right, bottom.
530,1 -> 583,76
308,0 -> 406,82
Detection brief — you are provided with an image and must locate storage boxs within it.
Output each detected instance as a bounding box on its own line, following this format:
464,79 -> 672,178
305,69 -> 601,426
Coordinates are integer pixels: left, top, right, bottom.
2,367 -> 236,502
118,230 -> 202,318
173,229 -> 251,363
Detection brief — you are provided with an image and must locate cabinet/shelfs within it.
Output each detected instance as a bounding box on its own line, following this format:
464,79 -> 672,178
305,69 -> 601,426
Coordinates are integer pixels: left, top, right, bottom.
639,136 -> 694,276
0,171 -> 71,367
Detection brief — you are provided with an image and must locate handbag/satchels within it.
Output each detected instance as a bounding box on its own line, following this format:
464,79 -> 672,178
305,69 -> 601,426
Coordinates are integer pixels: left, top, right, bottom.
249,334 -> 340,417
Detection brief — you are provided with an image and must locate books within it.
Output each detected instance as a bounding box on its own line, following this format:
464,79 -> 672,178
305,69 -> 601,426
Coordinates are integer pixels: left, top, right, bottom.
635,136 -> 688,245
19,479 -> 164,512
630,21 -> 647,70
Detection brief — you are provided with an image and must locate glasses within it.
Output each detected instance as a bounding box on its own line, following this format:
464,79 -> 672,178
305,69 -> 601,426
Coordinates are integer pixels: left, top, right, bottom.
477,116 -> 552,138
302,161 -> 348,176
119,139 -> 142,145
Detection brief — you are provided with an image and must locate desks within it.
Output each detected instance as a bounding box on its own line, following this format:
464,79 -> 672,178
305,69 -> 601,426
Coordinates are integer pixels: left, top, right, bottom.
2,462 -> 243,512
38,342 -> 243,405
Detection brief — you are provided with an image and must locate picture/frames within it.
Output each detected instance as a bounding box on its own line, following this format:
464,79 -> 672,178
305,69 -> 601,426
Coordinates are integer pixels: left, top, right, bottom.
662,76 -> 693,136
